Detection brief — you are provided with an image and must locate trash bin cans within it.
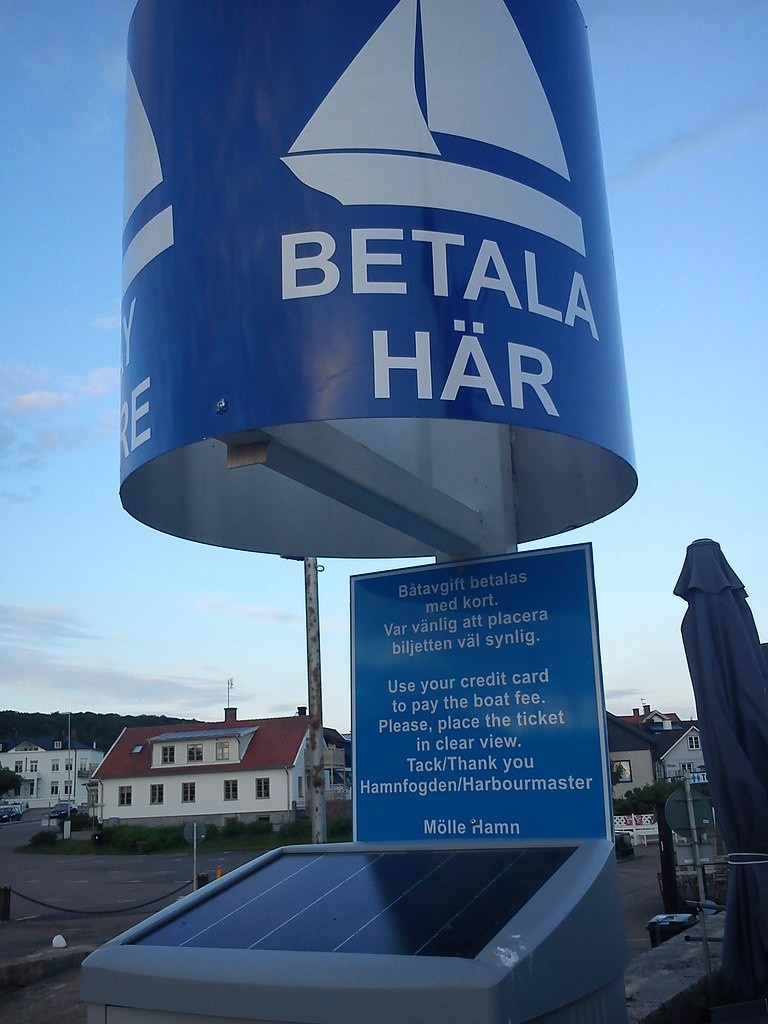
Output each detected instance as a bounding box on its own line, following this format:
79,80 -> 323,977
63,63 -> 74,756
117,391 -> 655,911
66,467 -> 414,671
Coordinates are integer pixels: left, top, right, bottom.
644,911 -> 699,950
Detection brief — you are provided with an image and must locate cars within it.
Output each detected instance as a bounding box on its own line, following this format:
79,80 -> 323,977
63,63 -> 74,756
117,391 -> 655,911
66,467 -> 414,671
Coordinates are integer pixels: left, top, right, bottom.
49,801 -> 79,818
0,805 -> 22,823
